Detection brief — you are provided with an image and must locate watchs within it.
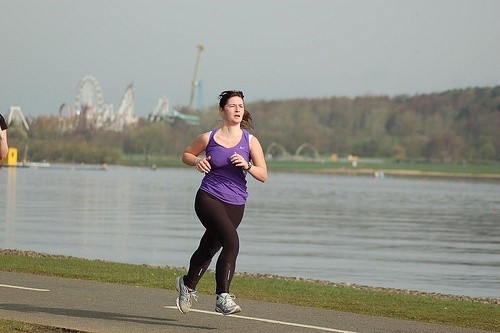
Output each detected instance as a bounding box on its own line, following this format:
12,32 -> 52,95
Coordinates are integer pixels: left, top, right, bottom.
246,162 -> 252,170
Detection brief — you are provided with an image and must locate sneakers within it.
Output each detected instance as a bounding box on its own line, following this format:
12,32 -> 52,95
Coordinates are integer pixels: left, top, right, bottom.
215,293 -> 242,316
175,274 -> 198,314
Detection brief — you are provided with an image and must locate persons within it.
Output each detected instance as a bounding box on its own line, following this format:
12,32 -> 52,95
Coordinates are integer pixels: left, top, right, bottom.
0,114 -> 8,167
175,89 -> 268,315
